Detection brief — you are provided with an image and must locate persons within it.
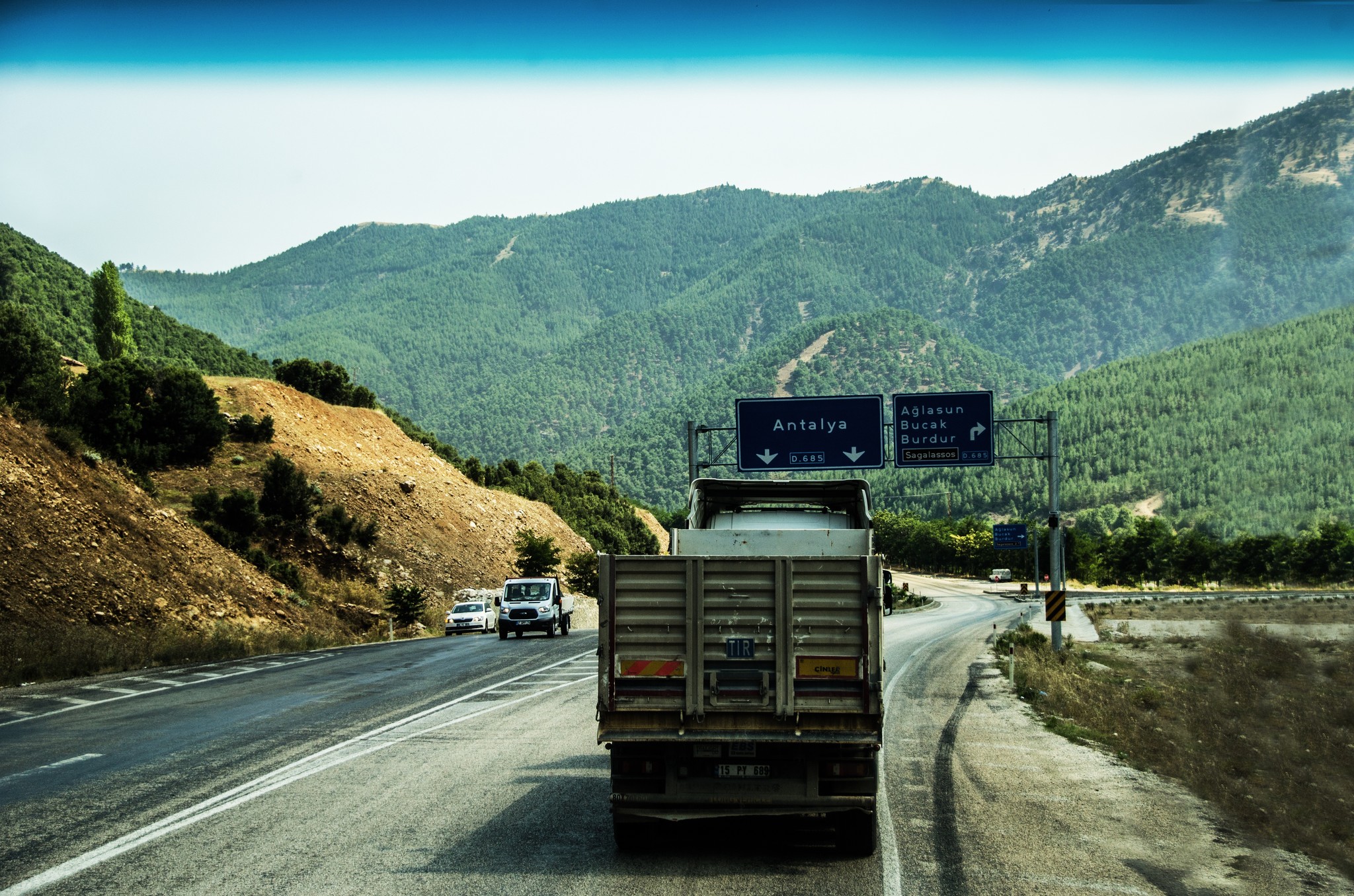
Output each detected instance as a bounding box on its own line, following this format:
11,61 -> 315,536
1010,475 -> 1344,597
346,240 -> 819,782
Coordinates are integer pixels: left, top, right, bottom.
536,587 -> 548,598
473,606 -> 480,612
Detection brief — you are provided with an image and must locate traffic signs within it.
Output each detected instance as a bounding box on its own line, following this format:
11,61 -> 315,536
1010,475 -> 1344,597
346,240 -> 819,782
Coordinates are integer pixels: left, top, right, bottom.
992,523 -> 1028,549
735,388 -> 996,473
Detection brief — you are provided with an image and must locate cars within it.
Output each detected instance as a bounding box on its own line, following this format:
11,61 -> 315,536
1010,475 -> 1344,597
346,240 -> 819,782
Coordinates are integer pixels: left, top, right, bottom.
445,601 -> 497,635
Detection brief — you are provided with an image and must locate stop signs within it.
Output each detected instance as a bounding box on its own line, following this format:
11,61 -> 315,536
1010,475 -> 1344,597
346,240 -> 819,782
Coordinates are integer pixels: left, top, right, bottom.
1044,575 -> 1049,580
995,576 -> 999,582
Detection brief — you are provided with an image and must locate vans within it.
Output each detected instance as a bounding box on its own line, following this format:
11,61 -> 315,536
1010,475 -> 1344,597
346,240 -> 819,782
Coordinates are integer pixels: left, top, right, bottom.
989,569 -> 1011,582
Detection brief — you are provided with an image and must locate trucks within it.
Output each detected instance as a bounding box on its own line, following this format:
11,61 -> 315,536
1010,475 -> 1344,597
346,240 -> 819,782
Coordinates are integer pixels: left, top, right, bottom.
495,574 -> 575,639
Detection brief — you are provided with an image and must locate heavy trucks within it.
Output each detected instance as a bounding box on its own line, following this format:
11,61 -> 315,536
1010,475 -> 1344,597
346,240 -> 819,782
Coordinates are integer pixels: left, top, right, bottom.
594,474 -> 894,852
